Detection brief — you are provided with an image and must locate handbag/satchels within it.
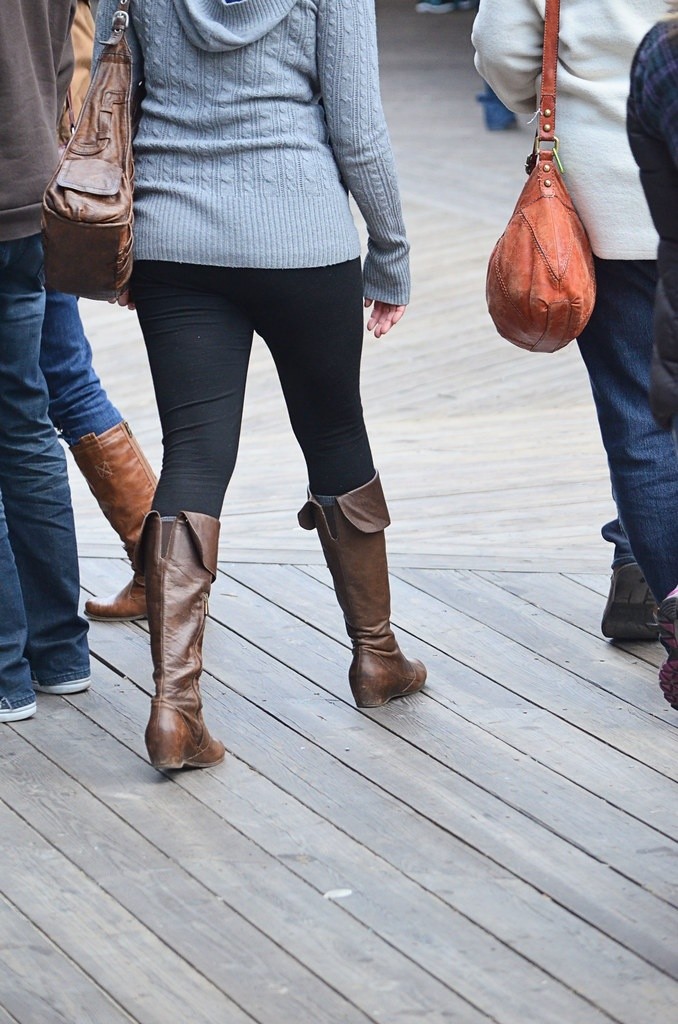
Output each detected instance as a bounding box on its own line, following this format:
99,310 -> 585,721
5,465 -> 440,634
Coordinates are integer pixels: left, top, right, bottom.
40,2 -> 136,302
484,141 -> 597,354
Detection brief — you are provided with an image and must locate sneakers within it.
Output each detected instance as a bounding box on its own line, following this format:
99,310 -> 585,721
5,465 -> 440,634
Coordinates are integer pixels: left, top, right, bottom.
30,674 -> 93,694
600,561 -> 660,643
656,585 -> 678,712
0,697 -> 37,723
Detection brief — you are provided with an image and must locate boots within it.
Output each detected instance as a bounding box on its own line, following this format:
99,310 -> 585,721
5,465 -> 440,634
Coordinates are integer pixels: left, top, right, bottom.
298,468 -> 427,708
68,420 -> 160,622
131,510 -> 226,772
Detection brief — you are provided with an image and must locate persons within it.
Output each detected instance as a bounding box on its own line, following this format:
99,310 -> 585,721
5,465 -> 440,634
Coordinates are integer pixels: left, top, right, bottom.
0,0 -> 91,729
100,0 -> 428,773
38,0 -> 163,620
415,1 -> 678,718
